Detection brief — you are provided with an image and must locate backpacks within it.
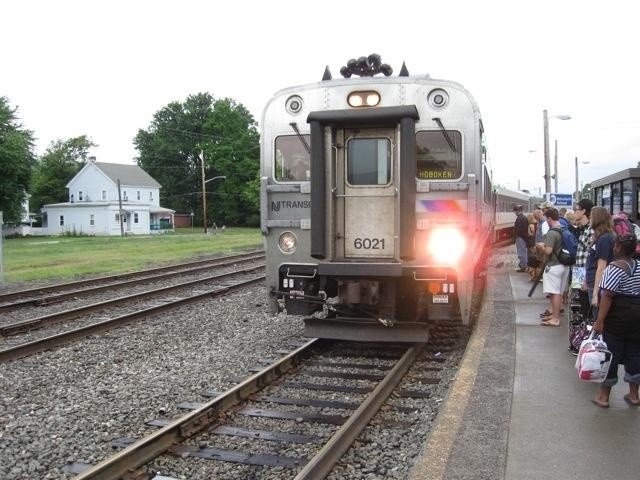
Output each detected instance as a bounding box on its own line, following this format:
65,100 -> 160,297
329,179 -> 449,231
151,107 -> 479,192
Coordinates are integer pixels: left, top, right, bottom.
556,226 -> 578,265
612,214 -> 640,255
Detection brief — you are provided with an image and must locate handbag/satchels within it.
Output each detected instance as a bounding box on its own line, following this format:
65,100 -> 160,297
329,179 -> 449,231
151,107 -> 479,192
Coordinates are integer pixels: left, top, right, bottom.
575,340 -> 613,383
570,320 -> 602,350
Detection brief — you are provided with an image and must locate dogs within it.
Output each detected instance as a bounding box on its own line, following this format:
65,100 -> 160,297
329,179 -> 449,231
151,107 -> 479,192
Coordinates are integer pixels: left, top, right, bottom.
522,267 -> 543,281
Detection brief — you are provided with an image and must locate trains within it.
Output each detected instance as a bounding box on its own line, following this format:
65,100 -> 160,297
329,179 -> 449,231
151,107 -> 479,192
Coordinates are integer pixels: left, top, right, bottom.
259,54 -> 544,342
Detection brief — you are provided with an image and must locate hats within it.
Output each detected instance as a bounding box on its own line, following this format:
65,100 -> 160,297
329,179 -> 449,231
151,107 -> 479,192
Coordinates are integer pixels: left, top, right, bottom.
513,205 -> 522,210
537,201 -> 553,209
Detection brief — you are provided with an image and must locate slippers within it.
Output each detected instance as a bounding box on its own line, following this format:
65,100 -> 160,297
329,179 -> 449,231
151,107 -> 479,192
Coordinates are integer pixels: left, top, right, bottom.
624,393 -> 640,406
540,309 -> 560,327
593,395 -> 610,407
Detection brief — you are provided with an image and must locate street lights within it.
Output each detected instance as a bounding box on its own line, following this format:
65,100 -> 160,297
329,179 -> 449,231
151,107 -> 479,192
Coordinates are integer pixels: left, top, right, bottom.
576,162 -> 590,191
528,151 -> 557,193
544,110 -> 572,193
202,176 -> 226,233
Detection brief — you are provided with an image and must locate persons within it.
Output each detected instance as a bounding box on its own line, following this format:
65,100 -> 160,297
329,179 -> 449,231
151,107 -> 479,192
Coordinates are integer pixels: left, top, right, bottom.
512,198 -> 640,354
591,231 -> 640,410
